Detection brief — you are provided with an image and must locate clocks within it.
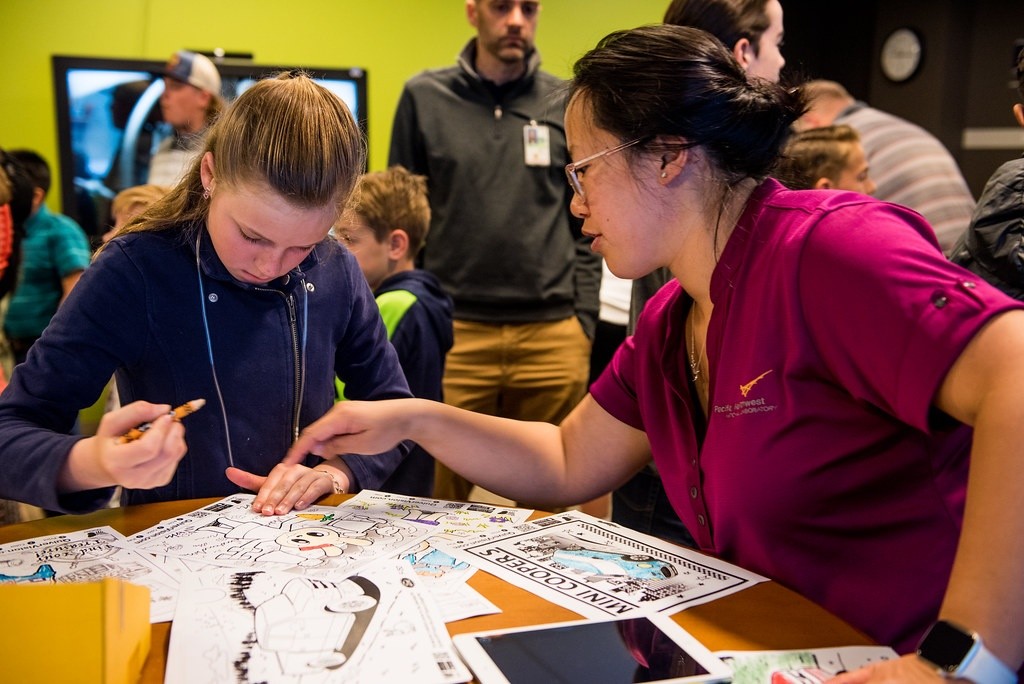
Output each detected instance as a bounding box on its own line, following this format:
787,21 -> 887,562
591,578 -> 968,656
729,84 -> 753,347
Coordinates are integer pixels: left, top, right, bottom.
877,29 -> 924,83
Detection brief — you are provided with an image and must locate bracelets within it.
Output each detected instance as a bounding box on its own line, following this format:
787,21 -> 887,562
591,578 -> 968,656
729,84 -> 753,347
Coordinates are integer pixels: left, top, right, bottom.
318,470 -> 344,494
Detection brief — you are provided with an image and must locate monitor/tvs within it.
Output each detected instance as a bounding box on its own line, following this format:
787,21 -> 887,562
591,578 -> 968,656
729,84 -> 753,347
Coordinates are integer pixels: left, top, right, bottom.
50,53 -> 369,249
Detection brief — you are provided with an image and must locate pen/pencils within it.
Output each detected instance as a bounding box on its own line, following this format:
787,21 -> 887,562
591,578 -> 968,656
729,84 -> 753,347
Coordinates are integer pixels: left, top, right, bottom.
115,397 -> 206,446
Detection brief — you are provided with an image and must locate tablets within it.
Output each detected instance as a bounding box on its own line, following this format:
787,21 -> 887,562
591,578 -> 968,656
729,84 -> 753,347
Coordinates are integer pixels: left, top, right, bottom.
452,611 -> 733,684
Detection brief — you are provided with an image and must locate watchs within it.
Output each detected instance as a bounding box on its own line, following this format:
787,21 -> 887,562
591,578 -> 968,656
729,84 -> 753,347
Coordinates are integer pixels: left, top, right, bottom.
916,618 -> 1019,684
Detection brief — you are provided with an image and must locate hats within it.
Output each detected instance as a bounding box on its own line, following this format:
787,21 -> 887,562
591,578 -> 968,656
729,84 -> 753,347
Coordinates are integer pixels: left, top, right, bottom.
148,52 -> 221,97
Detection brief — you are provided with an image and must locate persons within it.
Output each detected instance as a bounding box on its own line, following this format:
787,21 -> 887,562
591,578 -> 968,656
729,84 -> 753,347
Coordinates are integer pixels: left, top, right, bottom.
0,72 -> 416,518
0,146 -> 91,395
150,48 -> 226,191
790,78 -> 980,258
946,40 -> 1024,302
104,184 -> 171,242
330,167 -> 454,497
388,0 -> 614,527
281,23 -> 1024,684
617,0 -> 789,551
618,618 -> 709,684
775,123 -> 875,198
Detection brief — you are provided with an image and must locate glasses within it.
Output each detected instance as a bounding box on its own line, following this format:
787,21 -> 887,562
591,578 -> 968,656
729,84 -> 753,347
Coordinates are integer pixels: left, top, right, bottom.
565,131 -> 657,204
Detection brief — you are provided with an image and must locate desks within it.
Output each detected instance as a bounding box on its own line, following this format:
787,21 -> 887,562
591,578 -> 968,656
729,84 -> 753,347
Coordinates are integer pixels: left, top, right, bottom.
0,494 -> 885,684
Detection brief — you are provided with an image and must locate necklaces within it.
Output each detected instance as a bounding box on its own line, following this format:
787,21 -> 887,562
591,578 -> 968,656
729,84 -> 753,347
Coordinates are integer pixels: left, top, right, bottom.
193,228 -> 310,473
690,301 -> 705,380
